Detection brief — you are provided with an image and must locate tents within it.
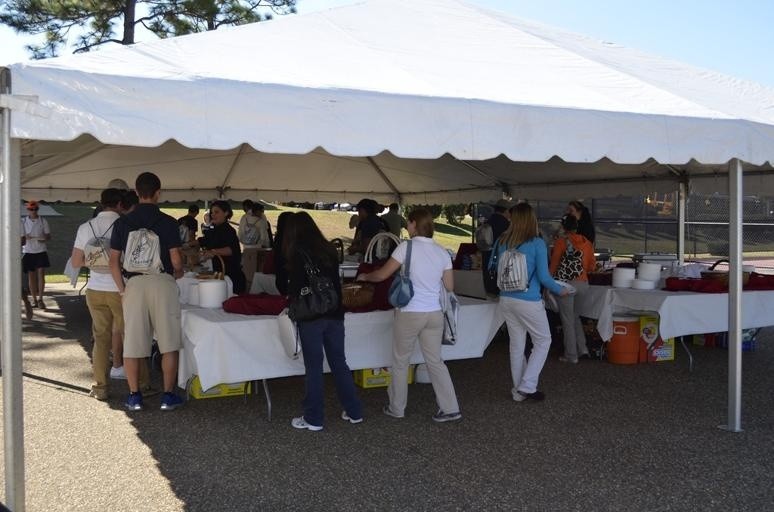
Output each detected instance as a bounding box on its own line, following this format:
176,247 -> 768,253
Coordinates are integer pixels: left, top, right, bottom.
0,1 -> 774,509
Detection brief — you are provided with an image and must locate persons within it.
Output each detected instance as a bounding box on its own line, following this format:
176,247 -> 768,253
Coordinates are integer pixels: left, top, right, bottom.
22,201 -> 50,309
201,211 -> 214,236
72,188 -> 161,400
348,199 -> 389,259
112,189 -> 138,379
190,200 -> 247,294
109,172 -> 185,412
380,203 -> 408,238
488,203 -> 570,402
542,214 -> 595,365
178,205 -> 200,246
20,220 -> 32,322
243,200 -> 274,248
567,199 -> 597,244
356,208 -> 462,423
92,178 -> 129,219
276,209 -> 365,432
238,203 -> 271,248
482,200 -> 510,298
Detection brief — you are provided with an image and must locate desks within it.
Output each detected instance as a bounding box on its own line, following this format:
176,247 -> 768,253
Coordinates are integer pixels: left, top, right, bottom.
177,295 -> 505,423
596,287 -> 774,373
579,284 -> 632,360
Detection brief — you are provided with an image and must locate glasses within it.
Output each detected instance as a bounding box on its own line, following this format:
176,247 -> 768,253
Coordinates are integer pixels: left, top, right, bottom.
27,207 -> 39,212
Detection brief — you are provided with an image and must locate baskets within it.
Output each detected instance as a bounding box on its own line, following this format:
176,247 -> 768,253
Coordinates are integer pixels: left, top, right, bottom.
340,282 -> 375,309
189,250 -> 225,280
701,269 -> 750,289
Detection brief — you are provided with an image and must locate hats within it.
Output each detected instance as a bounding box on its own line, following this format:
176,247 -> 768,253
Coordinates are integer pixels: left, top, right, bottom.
24,201 -> 40,208
106,176 -> 129,191
488,198 -> 513,211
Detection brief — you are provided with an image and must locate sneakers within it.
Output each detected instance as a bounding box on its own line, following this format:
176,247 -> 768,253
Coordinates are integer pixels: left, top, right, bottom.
341,411 -> 365,424
432,410 -> 463,421
87,386 -> 109,400
291,416 -> 324,431
124,392 -> 144,411
517,388 -> 546,401
579,350 -> 591,360
383,405 -> 405,419
37,301 -> 46,309
159,392 -> 185,411
559,355 -> 578,365
109,366 -> 129,381
31,299 -> 38,308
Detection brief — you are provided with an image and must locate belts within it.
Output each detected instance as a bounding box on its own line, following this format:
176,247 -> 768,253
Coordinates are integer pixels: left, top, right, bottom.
124,272 -> 145,279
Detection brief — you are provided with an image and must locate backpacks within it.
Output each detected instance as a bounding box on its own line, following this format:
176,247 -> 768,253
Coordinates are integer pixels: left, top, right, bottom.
84,219 -> 118,274
496,234 -> 539,292
121,212 -> 168,275
376,228 -> 390,260
240,217 -> 262,245
474,223 -> 494,251
178,217 -> 193,242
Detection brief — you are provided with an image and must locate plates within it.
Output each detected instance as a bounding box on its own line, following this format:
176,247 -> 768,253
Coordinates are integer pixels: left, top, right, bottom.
189,282 -> 200,305
198,280 -> 228,309
612,267 -> 636,288
638,264 -> 661,280
634,280 -> 654,290
555,281 -> 576,293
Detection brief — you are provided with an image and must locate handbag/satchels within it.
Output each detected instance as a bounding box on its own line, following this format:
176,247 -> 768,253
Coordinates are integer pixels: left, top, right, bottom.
355,230 -> 403,311
553,237 -> 585,284
281,249 -> 337,323
482,237 -> 501,299
385,239 -> 416,307
